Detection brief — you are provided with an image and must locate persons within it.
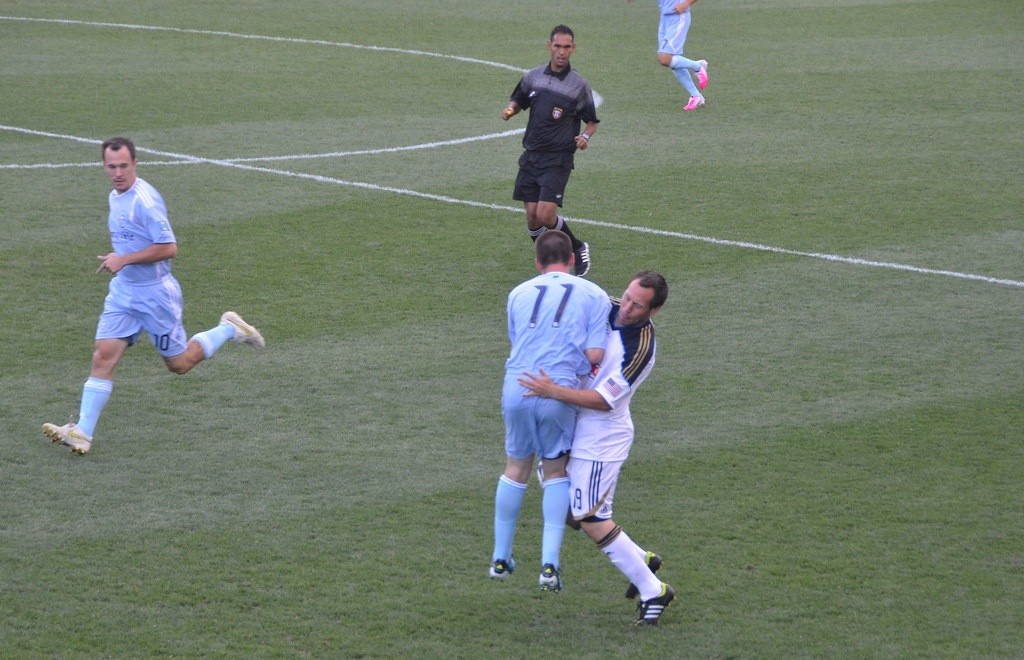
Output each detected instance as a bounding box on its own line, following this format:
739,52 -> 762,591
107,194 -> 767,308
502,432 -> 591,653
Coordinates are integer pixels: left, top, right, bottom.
487,227 -> 676,633
42,137 -> 266,457
656,0 -> 709,112
500,22 -> 603,277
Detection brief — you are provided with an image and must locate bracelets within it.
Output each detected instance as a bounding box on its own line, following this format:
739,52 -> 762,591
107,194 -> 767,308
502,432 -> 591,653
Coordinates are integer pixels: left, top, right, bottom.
581,131 -> 591,143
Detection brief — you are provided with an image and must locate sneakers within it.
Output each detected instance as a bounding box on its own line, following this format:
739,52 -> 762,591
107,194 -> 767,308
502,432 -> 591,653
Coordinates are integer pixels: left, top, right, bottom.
634,583 -> 675,627
490,559 -> 514,581
539,563 -> 561,592
695,60 -> 709,89
574,242 -> 590,277
625,552 -> 662,599
42,422 -> 92,455
682,93 -> 706,111
219,311 -> 265,348
